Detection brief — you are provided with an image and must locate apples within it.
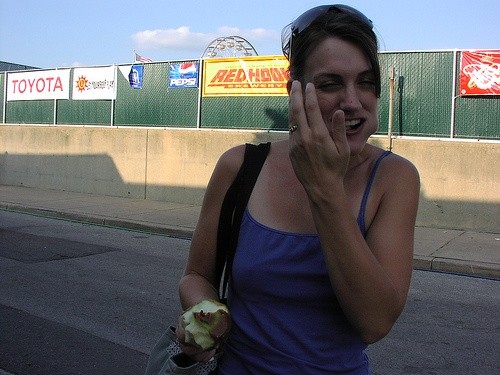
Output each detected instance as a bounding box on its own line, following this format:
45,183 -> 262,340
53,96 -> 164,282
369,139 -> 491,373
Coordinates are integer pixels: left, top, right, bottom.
180,298 -> 230,350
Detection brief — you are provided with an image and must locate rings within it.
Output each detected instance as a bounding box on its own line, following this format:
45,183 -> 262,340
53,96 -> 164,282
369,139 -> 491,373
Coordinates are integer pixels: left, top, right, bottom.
288,121 -> 299,134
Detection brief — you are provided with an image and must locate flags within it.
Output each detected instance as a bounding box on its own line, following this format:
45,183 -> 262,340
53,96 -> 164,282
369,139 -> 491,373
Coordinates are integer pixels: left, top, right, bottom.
134,52 -> 152,63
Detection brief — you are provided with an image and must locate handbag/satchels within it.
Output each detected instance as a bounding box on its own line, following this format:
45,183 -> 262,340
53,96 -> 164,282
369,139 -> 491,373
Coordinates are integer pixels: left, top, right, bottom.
144,298 -> 227,375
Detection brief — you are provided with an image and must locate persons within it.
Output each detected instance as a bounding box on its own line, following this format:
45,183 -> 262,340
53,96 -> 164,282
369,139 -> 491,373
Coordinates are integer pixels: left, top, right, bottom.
179,5 -> 421,375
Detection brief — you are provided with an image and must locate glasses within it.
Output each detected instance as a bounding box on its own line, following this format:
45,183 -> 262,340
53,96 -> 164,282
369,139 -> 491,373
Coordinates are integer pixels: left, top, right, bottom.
289,4 -> 373,69
